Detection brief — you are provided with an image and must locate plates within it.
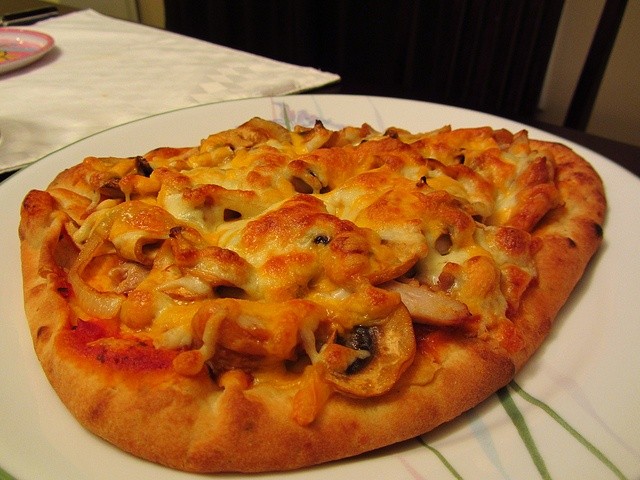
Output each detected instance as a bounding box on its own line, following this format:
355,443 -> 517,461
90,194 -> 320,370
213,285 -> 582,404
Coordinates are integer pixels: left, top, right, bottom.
0,27 -> 55,76
